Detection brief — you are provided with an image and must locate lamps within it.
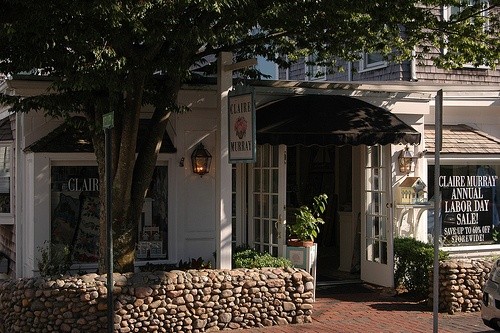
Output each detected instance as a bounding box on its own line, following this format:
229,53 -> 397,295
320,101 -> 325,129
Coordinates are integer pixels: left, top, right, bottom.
190,143 -> 213,178
398,145 -> 413,176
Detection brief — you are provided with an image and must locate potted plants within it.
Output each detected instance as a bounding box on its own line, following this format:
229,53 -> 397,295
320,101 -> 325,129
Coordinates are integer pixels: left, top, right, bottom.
286,194 -> 328,247
